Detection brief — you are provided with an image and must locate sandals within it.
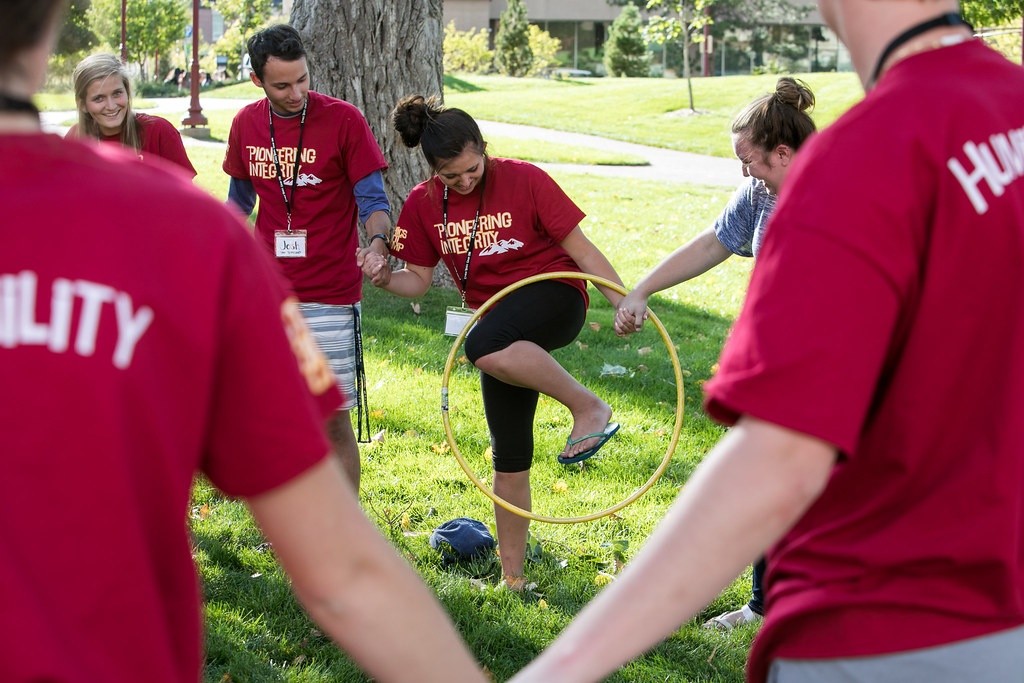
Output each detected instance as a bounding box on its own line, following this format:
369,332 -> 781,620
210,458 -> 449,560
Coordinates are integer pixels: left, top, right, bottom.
703,602 -> 764,632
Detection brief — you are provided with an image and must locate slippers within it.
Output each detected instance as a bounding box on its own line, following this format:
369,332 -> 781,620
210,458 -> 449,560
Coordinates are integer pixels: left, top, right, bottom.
558,422 -> 620,464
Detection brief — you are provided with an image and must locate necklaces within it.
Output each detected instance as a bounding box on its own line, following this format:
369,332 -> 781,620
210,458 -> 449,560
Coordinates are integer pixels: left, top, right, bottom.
867,14 -> 975,95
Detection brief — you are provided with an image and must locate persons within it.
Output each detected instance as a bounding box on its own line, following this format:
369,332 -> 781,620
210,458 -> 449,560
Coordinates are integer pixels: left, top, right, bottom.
64,54 -> 197,186
614,77 -> 819,336
223,24 -> 393,503
512,0 -> 1024,683
355,94 -> 649,594
0,0 -> 491,683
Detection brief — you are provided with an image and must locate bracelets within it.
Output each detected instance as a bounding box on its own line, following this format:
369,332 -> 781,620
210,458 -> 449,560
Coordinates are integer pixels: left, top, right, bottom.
368,233 -> 390,250
